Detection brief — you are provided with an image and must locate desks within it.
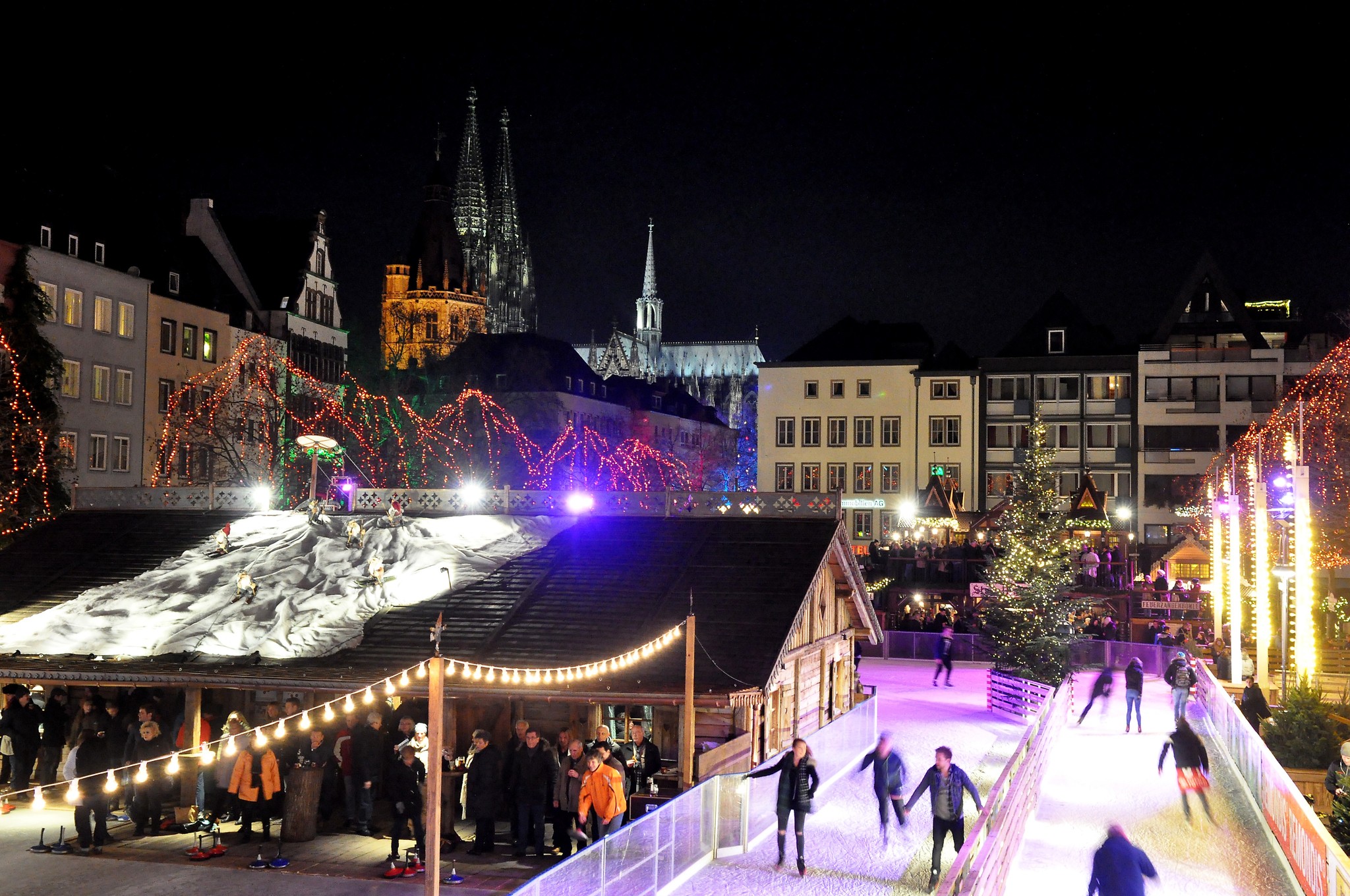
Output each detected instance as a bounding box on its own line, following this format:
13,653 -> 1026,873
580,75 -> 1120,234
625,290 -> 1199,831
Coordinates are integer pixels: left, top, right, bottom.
652,767 -> 678,790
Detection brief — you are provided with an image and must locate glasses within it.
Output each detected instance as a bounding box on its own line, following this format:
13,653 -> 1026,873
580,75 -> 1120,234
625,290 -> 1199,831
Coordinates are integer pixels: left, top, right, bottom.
526,737 -> 538,741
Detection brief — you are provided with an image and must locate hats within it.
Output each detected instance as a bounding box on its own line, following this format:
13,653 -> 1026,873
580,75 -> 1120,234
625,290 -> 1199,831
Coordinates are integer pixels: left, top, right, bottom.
414,722 -> 428,734
1176,651 -> 1186,658
2,683 -> 19,695
51,688 -> 67,697
1158,569 -> 1166,575
1340,742 -> 1350,757
16,685 -> 30,700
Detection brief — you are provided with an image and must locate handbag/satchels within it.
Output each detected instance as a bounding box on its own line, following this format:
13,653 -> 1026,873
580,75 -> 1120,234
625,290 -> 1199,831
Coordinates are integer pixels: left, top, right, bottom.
1255,704 -> 1272,720
0,735 -> 14,756
807,798 -> 818,814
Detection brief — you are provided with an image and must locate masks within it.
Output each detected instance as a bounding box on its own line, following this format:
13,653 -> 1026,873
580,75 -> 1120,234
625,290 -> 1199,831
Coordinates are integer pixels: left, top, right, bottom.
230,723 -> 240,729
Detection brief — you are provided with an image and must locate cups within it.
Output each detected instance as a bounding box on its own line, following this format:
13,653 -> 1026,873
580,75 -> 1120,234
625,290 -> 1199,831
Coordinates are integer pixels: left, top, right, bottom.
448,761 -> 455,770
646,777 -> 653,790
458,756 -> 465,766
649,784 -> 658,797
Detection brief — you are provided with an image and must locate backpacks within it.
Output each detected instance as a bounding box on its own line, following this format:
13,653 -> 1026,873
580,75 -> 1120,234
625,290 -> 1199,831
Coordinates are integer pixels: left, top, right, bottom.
1175,664 -> 1190,687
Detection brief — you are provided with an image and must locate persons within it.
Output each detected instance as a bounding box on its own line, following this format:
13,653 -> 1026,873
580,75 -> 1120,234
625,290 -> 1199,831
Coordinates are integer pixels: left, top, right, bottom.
549,727 -> 575,854
1067,537 -> 1288,670
745,738 -> 819,877
866,535 -> 1011,662
902,745 -> 984,890
1089,825 -> 1156,896
389,745 -> 426,859
854,640 -> 863,671
1163,651 -> 1198,722
457,729 -> 498,855
594,740 -> 626,803
1240,675 -> 1265,737
0,679 -> 361,851
578,749 -> 627,881
1324,740 -> 1350,823
509,727 -> 558,858
1158,717 -> 1210,816
1077,662 -> 1116,724
620,725 -> 662,823
390,715 -> 428,840
553,739 -> 588,861
1125,657 -> 1144,733
1217,649 -> 1232,680
857,731 -> 905,839
351,712 -> 385,836
931,626 -> 955,688
1240,648 -> 1255,680
582,724 -> 628,844
502,720 -> 531,846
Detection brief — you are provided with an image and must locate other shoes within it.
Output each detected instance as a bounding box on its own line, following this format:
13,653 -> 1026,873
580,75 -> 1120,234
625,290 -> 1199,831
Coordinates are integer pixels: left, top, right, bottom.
80,801 -> 283,851
927,867 -> 941,894
1125,726 -> 1141,734
932,680 -> 953,688
0,777 -> 59,803
796,856 -> 805,878
777,855 -> 785,866
317,821 -> 572,863
1078,717 -> 1084,724
